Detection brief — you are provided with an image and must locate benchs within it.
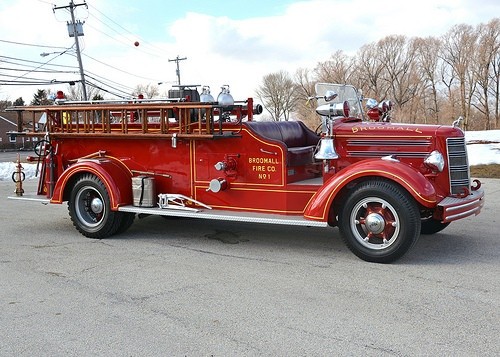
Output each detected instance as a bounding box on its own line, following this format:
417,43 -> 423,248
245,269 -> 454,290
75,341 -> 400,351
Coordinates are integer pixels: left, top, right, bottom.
246,121 -> 322,166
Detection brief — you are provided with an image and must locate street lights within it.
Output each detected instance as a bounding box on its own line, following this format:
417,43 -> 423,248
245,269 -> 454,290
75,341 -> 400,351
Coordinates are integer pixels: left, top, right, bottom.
39,51 -> 86,100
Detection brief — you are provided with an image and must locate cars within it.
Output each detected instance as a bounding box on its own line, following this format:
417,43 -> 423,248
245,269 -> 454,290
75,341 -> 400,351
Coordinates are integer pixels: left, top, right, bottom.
4,79 -> 486,264
